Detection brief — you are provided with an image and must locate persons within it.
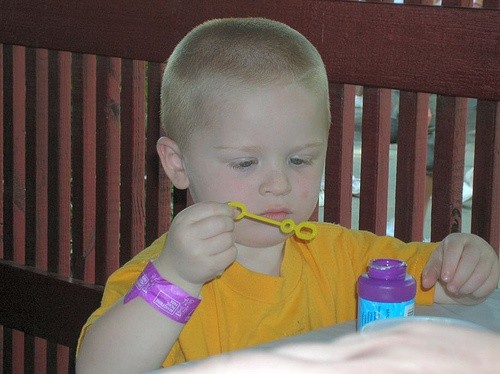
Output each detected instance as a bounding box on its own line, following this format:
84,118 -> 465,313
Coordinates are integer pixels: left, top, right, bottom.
74,16 -> 500,374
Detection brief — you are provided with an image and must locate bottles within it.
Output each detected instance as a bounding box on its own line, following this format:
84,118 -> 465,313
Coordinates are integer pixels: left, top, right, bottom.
355,258 -> 417,333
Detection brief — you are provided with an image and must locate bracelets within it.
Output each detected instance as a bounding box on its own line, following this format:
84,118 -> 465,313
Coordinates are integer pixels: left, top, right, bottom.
123,260 -> 202,325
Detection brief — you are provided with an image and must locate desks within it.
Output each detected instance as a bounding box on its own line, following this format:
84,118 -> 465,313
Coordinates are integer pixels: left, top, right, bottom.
147,279 -> 500,374
